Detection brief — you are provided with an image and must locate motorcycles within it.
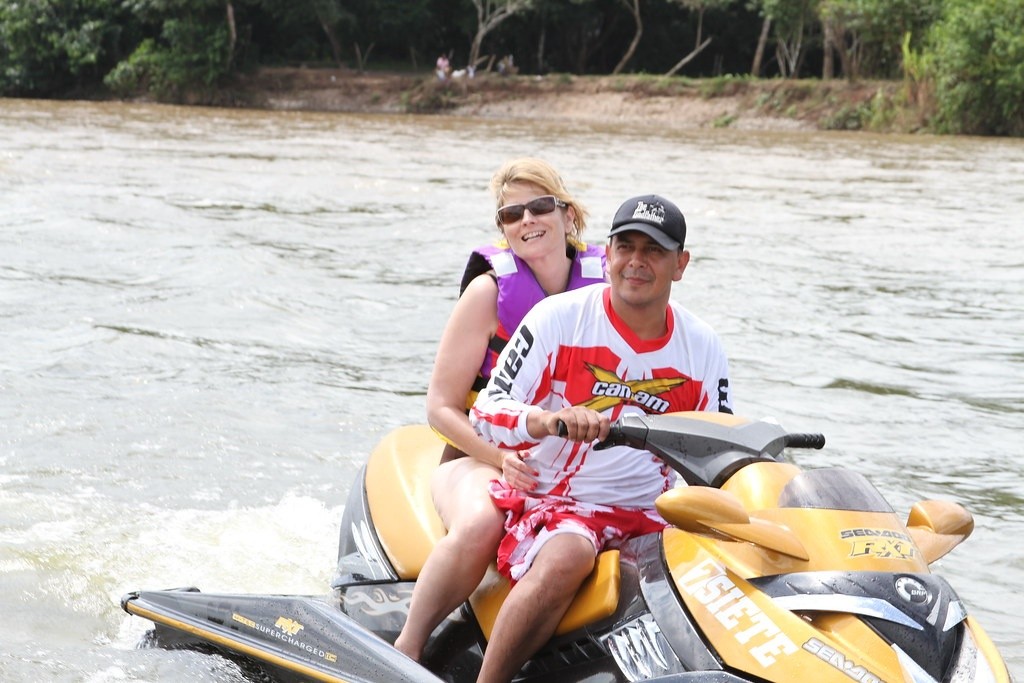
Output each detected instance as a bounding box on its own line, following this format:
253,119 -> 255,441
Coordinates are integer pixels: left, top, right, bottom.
124,410 -> 1012,683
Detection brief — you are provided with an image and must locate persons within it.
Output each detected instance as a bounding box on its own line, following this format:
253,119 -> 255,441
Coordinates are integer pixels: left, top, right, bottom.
469,194 -> 735,683
395,158 -> 611,664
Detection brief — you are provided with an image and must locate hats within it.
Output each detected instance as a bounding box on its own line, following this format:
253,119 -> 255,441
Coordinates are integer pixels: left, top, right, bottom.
608,195 -> 686,250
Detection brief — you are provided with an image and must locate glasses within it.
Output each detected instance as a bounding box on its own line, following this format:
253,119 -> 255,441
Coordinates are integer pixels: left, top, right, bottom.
497,194 -> 568,225
